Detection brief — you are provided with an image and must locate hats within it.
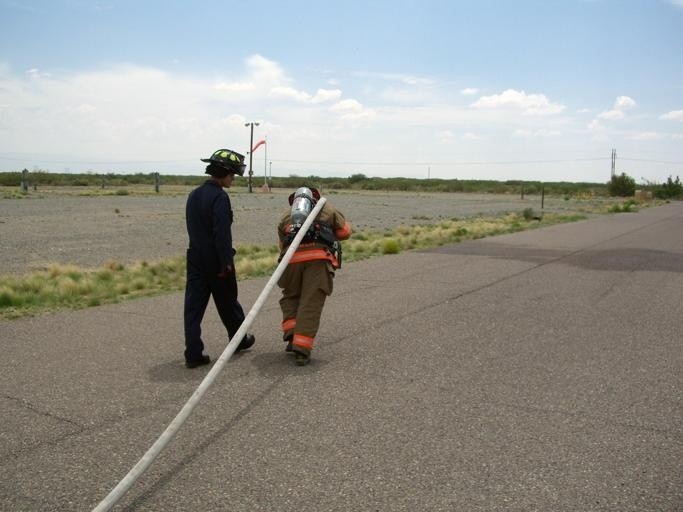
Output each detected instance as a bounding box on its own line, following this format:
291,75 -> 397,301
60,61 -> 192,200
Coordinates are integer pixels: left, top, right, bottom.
288,186 -> 321,207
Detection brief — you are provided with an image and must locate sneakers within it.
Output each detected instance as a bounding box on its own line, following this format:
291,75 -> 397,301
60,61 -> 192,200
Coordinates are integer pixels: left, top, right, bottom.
285,335 -> 311,366
185,355 -> 210,369
233,333 -> 255,353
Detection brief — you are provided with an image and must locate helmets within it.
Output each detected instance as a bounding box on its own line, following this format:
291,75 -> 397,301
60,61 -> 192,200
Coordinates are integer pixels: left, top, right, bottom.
200,149 -> 248,177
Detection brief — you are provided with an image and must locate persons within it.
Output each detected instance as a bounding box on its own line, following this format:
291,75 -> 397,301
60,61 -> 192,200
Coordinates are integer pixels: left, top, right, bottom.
182,148 -> 256,370
273,184 -> 351,367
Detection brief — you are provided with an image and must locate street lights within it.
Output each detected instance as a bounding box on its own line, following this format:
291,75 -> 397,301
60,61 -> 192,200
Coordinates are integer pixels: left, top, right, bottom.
243,121 -> 260,193
